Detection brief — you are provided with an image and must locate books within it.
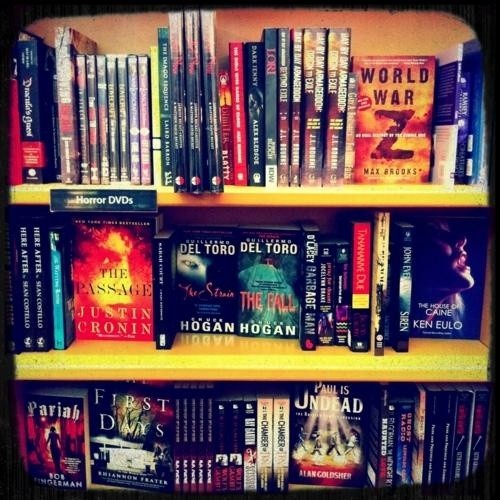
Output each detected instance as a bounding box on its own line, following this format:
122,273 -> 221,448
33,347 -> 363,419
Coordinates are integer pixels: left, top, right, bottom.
7,208 -> 489,353
8,9 -> 488,195
14,383 -> 494,496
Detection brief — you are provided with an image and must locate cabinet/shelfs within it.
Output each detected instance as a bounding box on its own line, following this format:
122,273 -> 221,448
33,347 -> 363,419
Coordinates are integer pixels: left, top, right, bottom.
9,179 -> 492,385
